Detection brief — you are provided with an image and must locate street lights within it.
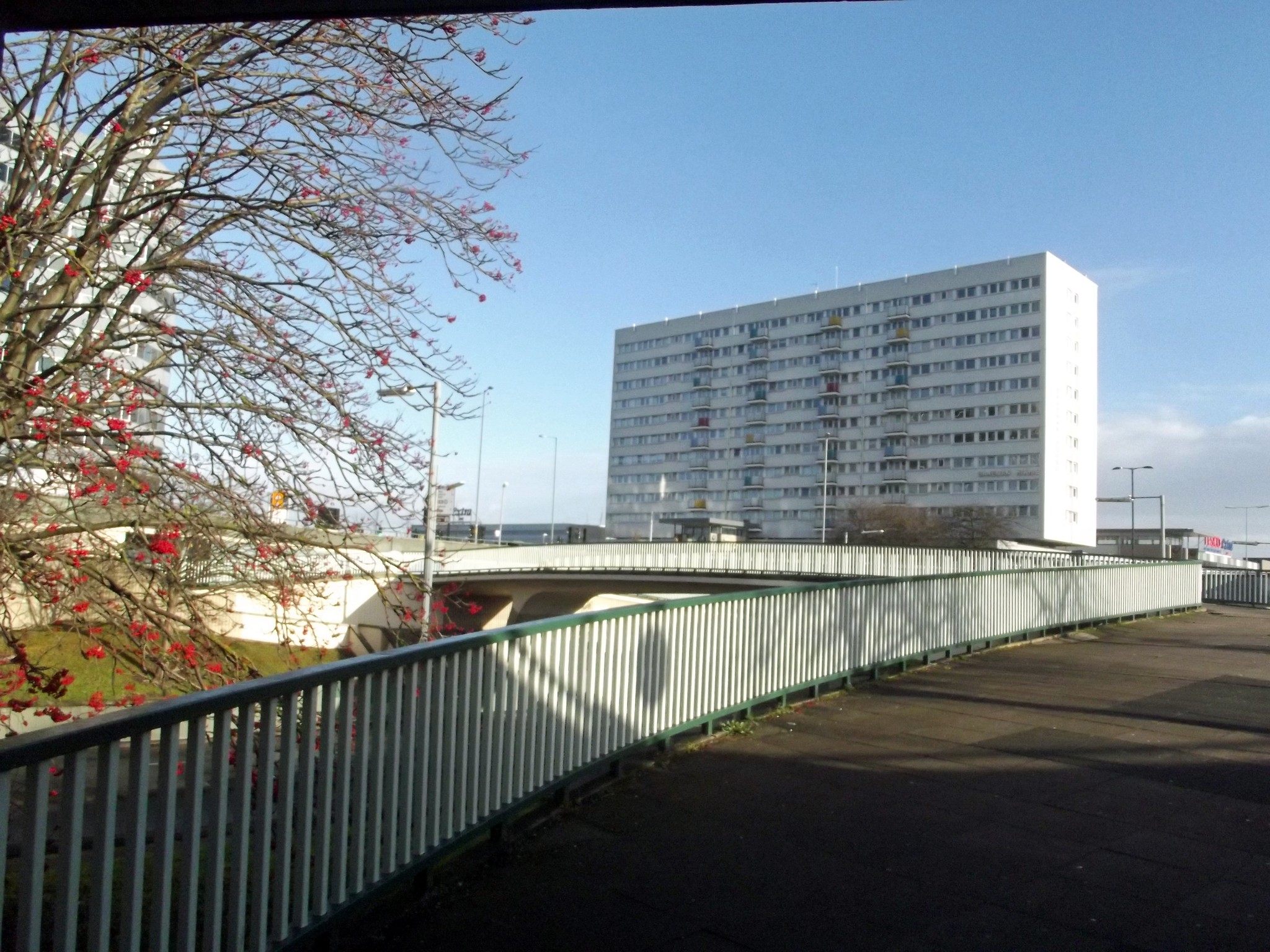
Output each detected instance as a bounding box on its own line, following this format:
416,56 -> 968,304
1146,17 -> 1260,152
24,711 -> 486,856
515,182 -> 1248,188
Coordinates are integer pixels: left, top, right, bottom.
1224,506 -> 1270,568
539,434 -> 558,545
376,380 -> 441,643
1112,466 -> 1154,564
474,386 -> 494,546
816,435 -> 840,543
